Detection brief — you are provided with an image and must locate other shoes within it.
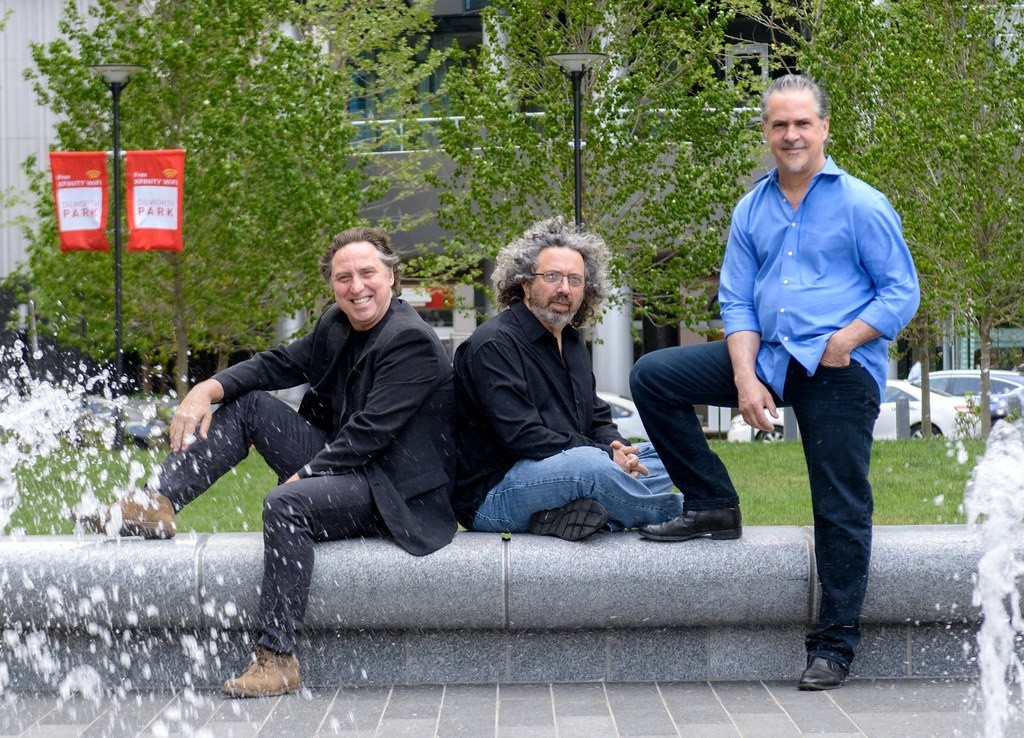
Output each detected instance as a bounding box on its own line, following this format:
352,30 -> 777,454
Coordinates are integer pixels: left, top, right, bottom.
529,499 -> 608,541
73,492 -> 176,540
225,649 -> 302,697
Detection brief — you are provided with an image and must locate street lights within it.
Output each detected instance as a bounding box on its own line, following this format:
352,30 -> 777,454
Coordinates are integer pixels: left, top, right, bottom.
546,50 -> 610,235
90,62 -> 147,401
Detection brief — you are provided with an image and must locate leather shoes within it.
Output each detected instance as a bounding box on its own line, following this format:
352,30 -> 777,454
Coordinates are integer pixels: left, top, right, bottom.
799,655 -> 848,690
639,503 -> 742,541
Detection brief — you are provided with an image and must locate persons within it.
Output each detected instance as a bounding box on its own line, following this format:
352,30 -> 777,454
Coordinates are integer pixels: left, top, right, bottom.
70,227 -> 458,697
450,215 -> 684,541
629,76 -> 920,690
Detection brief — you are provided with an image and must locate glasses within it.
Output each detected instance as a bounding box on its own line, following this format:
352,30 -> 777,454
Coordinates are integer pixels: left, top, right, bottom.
533,271 -> 585,287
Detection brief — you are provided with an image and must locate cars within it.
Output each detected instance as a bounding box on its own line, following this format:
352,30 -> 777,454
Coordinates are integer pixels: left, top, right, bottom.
595,390 -> 651,444
909,366 -> 1024,426
727,376 -> 983,445
